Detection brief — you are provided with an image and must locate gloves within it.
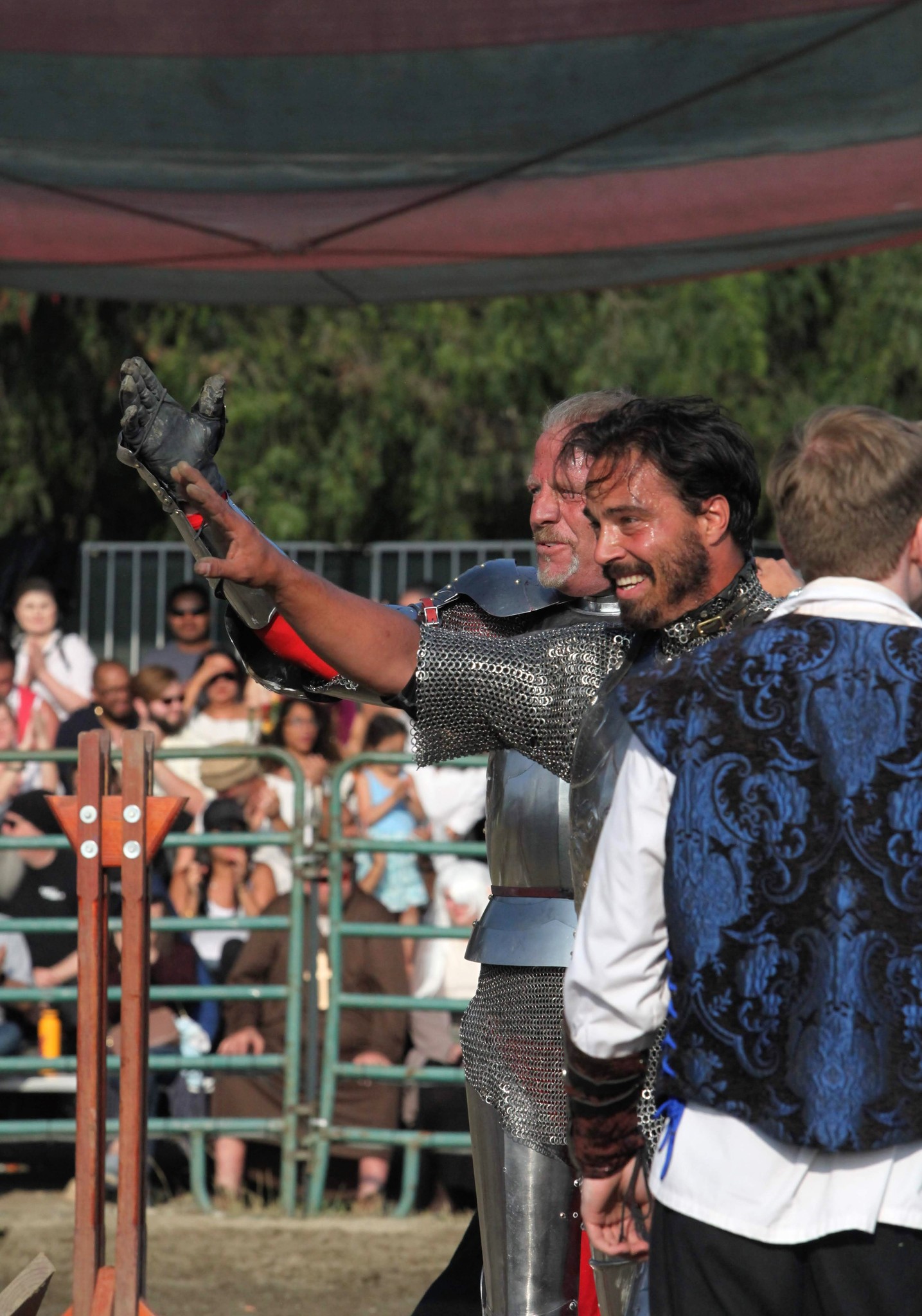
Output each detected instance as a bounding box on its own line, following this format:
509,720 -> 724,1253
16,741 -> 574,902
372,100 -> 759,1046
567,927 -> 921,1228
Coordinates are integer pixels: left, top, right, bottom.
116,356 -> 299,630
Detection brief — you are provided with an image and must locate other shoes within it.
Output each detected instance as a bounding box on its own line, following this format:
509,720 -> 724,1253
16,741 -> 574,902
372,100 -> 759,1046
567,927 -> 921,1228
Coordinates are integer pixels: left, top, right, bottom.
104,1148 -> 120,1190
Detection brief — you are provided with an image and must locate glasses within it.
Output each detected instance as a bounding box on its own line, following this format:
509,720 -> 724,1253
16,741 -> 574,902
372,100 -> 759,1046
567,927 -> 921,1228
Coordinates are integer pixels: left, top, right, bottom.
160,694 -> 185,705
167,605 -> 207,617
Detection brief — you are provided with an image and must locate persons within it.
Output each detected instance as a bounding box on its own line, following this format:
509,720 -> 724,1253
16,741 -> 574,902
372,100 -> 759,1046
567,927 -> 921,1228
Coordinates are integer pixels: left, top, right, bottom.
0,580 -> 488,1217
110,356 -> 804,1316
563,407 -> 922,1316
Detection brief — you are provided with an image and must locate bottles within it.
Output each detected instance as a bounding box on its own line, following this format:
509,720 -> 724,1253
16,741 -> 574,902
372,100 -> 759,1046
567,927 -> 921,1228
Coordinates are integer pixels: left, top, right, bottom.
38,1008 -> 62,1074
